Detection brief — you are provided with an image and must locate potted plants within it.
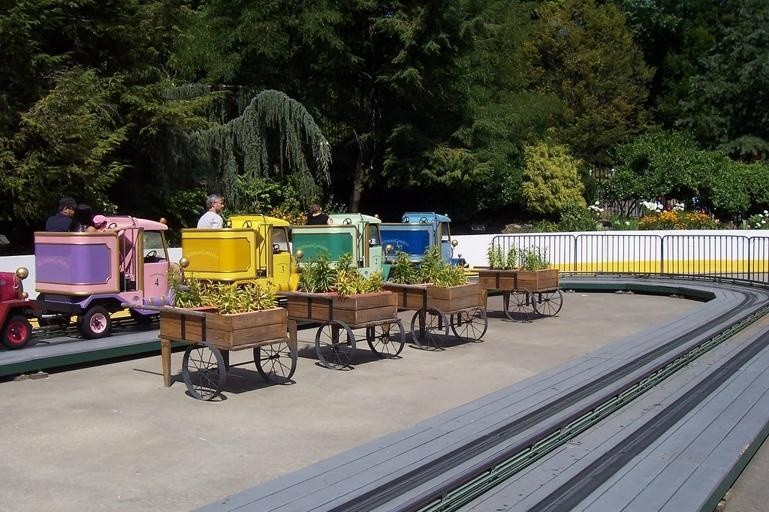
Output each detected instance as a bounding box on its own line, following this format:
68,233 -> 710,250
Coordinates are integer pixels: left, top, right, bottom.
382,244 -> 482,312
473,241 -> 558,291
160,269 -> 287,347
276,252 -> 398,325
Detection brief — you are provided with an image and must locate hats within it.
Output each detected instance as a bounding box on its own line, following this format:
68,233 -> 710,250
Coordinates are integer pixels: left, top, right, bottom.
58,197 -> 77,212
93,215 -> 111,227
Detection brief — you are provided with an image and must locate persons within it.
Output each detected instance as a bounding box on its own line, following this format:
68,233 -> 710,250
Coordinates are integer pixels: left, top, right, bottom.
69,203 -> 96,233
46,196 -> 76,232
93,214 -> 111,233
306,203 -> 334,226
196,193 -> 226,229
